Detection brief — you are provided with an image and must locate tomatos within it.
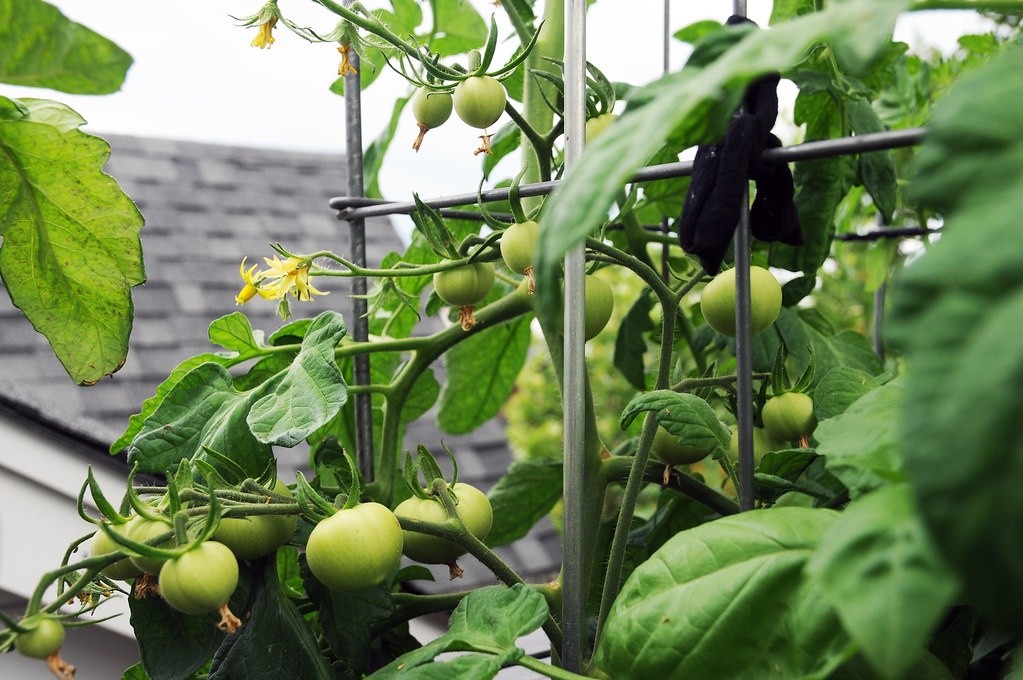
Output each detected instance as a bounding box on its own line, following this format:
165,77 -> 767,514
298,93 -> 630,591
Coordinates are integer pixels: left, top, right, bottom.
11,73 -> 819,679
